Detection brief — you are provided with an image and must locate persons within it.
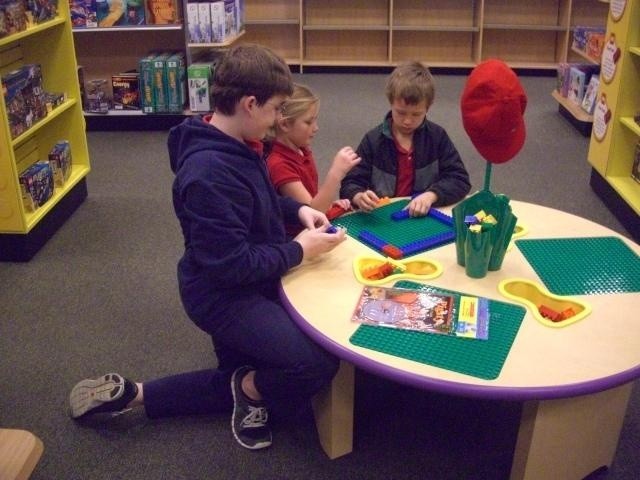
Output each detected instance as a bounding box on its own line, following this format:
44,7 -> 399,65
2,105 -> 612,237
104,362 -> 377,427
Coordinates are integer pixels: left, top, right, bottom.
70,43 -> 348,450
339,60 -> 472,218
265,83 -> 362,237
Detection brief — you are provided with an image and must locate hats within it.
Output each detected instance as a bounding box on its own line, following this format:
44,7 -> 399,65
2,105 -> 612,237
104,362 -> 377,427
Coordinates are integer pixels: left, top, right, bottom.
461,59 -> 528,164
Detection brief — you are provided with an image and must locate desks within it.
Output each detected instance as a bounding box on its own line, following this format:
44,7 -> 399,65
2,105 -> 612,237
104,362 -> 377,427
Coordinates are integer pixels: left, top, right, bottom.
273,193 -> 639,479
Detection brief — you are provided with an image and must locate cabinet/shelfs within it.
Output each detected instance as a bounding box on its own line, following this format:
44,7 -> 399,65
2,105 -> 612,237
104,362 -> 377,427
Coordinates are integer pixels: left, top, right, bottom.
1,0 -> 92,237
244,1 -> 610,74
549,1 -> 639,218
67,0 -> 244,119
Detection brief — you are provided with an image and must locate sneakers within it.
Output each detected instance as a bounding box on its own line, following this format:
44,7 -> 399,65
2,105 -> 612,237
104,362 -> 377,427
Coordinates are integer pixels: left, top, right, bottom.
68,372 -> 139,419
230,365 -> 273,450
